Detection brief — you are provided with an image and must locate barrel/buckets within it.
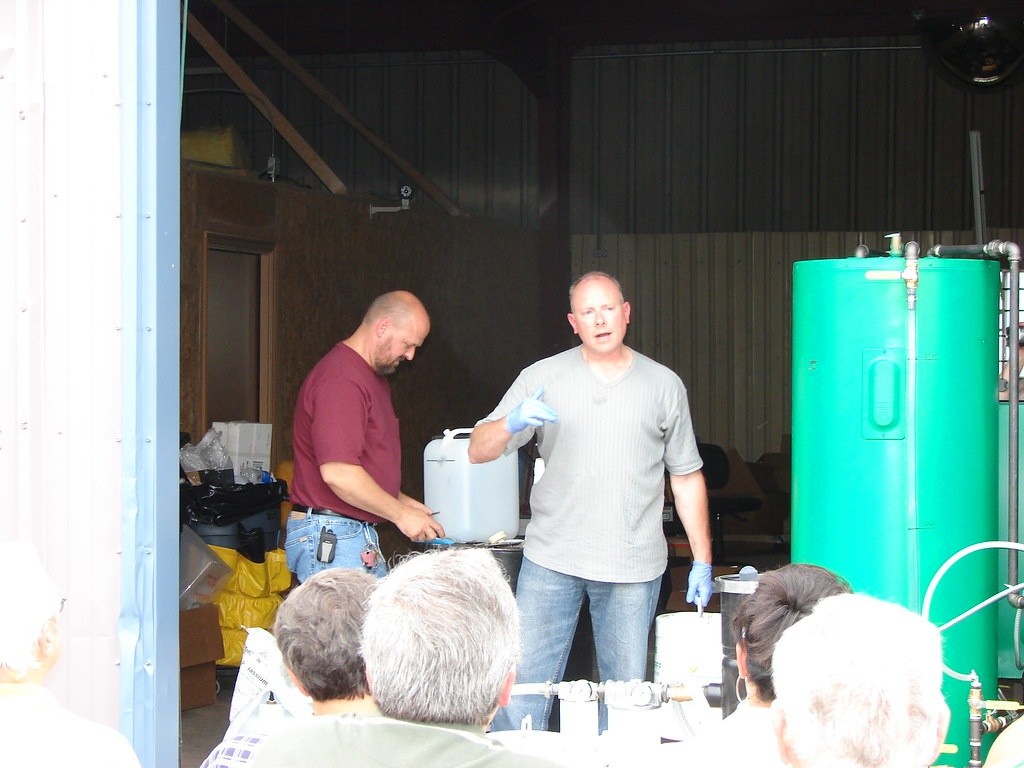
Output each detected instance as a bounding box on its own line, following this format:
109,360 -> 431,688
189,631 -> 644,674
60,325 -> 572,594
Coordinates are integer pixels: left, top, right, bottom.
425,427 -> 520,541
654,597 -> 723,688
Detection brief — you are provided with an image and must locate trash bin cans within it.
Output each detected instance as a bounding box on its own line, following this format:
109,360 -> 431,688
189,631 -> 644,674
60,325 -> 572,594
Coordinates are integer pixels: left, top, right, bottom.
179,479 -> 287,563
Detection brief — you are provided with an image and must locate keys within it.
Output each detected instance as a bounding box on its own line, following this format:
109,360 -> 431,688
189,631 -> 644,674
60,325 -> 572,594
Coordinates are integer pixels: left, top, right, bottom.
361,547 -> 386,570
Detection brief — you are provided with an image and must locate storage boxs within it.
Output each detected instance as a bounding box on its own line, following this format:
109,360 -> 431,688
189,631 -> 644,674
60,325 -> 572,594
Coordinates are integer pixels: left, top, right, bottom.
211,422 -> 274,486
179,604 -> 224,711
179,525 -> 235,609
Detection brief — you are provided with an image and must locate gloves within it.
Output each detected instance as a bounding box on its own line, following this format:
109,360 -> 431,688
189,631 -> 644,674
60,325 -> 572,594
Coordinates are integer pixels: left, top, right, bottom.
686,561 -> 713,607
506,387 -> 558,434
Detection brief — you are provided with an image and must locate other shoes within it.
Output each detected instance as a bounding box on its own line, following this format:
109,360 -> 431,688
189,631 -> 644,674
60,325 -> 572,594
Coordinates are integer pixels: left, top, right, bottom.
186,482 -> 279,527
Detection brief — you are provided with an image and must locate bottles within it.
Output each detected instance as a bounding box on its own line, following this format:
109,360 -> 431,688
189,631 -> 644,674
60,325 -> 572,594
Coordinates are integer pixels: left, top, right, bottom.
240,468 -> 273,485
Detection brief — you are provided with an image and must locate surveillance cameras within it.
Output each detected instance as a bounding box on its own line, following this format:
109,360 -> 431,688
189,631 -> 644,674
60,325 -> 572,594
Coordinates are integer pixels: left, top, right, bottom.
398,184 -> 415,201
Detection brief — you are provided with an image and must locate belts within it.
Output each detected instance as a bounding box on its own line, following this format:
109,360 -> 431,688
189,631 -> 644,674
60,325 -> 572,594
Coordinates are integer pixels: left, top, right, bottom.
291,504 -> 379,527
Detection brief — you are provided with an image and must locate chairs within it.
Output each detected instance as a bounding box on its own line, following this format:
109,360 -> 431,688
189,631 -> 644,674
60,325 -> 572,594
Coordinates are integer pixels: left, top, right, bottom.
696,443 -> 760,566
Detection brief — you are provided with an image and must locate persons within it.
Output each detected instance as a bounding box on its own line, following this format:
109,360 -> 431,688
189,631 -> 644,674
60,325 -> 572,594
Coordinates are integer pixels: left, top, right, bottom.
468,269 -> 713,731
196,563 -> 377,767
247,548 -> 546,767
691,561 -> 951,768
286,291 -> 445,582
